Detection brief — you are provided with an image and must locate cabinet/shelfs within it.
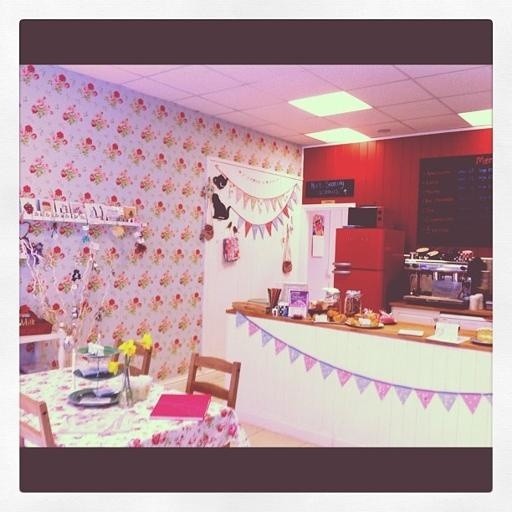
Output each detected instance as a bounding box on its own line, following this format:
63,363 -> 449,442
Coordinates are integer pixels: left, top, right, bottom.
438,312 -> 493,329
392,307 -> 438,324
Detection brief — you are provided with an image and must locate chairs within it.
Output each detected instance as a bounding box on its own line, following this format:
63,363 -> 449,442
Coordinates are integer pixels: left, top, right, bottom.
184,353 -> 242,447
113,337 -> 151,376
20,389 -> 54,448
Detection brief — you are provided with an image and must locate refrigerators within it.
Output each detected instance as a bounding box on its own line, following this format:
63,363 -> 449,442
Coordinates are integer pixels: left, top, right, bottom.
331,228 -> 406,315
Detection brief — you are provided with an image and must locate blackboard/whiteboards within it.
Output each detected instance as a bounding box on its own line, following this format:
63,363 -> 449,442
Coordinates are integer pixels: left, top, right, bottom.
416,154 -> 493,245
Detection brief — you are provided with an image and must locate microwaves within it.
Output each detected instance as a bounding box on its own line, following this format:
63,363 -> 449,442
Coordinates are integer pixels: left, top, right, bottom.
347,206 -> 394,230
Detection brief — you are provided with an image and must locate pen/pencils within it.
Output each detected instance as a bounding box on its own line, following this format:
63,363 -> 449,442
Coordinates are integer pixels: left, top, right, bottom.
268,289 -> 281,306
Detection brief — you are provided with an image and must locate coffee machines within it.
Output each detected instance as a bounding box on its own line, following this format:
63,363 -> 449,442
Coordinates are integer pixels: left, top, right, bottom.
401,258 -> 480,306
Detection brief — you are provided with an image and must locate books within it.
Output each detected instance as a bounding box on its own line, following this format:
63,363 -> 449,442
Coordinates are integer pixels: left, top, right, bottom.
149,393 -> 211,421
20,197 -> 137,221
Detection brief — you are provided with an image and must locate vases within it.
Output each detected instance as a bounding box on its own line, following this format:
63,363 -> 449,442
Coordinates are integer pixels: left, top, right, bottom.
117,366 -> 133,406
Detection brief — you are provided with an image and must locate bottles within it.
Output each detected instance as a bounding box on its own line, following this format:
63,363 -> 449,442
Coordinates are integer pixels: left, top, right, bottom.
343,291 -> 361,318
326,292 -> 340,312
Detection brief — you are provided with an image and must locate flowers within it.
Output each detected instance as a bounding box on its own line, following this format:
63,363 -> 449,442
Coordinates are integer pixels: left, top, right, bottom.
107,332 -> 153,405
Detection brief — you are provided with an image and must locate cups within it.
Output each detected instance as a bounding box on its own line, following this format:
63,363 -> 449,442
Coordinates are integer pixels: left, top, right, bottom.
136,374 -> 153,401
409,248 -> 473,262
469,292 -> 483,310
484,302 -> 492,310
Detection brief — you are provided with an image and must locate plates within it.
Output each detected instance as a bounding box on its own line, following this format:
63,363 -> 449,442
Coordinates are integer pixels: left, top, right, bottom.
73,366 -> 123,382
68,387 -> 117,406
78,345 -> 120,358
470,336 -> 493,346
425,332 -> 471,346
345,319 -> 384,329
